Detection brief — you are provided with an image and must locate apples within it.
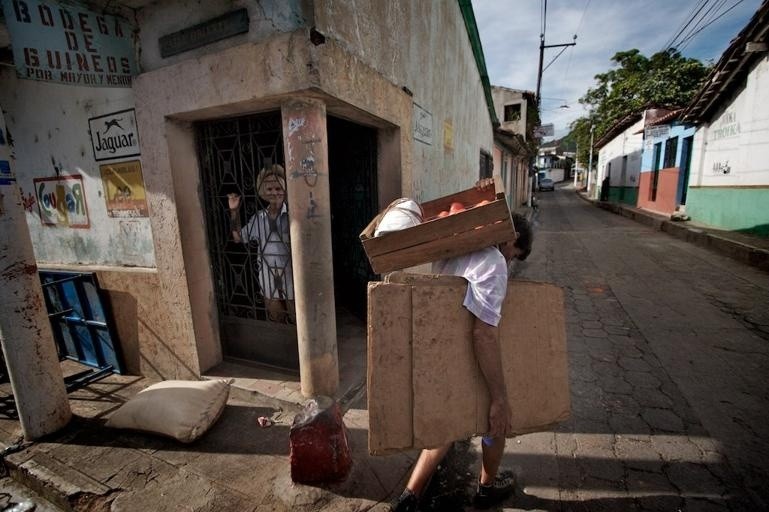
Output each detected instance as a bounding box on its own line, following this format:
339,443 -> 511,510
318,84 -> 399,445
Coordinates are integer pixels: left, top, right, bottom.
436,199 -> 505,231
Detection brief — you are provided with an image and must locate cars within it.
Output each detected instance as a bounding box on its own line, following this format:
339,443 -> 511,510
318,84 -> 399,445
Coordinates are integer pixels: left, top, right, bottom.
539,179 -> 555,190
571,169 -> 583,175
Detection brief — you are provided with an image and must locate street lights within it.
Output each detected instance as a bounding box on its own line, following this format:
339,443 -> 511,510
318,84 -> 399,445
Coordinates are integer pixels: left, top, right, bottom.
526,103 -> 571,207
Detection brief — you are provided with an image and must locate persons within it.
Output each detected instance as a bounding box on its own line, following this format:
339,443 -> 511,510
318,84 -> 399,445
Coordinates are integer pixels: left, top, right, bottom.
227,164 -> 295,324
531,192 -> 539,211
672,206 -> 688,221
389,210 -> 534,512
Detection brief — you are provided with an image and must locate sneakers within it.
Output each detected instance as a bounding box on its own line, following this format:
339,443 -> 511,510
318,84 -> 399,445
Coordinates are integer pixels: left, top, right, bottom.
476,470 -> 518,500
389,487 -> 431,511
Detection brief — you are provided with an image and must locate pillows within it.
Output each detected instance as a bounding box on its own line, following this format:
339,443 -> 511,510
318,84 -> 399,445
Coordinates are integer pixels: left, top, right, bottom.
104,378 -> 235,443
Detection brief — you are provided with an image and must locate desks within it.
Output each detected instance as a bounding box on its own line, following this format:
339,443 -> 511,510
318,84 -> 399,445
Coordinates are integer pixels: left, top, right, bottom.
0,265 -> 127,418
